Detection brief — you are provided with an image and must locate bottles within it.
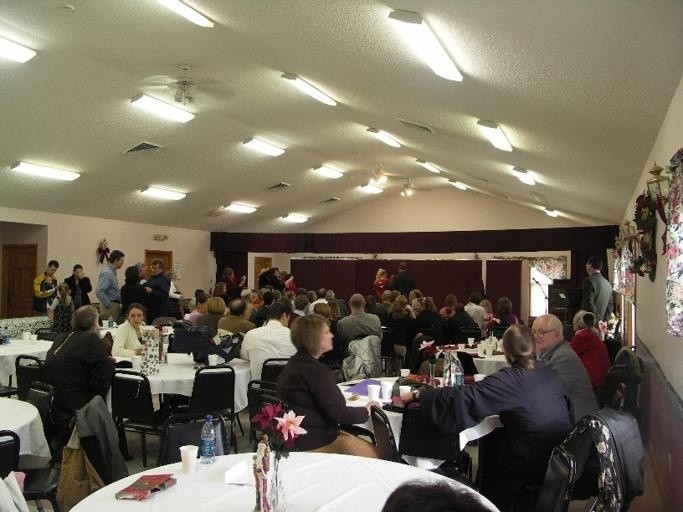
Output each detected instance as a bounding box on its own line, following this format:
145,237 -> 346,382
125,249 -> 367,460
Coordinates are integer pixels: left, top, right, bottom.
3,327 -> 9,346
201,415 -> 215,464
109,313 -> 113,327
454,361 -> 464,385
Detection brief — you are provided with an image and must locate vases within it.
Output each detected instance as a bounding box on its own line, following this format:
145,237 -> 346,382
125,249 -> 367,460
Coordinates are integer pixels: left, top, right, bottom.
267,474 -> 286,512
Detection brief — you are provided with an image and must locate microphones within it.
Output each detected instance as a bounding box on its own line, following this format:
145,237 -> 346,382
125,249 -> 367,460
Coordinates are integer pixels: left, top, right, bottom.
532,277 -> 538,286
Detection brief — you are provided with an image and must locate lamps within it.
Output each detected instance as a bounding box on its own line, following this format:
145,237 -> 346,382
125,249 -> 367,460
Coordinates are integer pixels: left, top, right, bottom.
415,158 -> 439,175
158,0 -> 214,29
140,186 -> 186,201
545,208 -> 556,218
366,127 -> 401,149
400,184 -> 413,198
281,216 -> 308,223
514,167 -> 536,186
281,72 -> 336,106
224,204 -> 257,215
614,160 -> 669,251
313,166 -> 343,179
388,10 -> 462,81
128,93 -> 196,123
477,120 -> 512,153
2,37 -> 37,63
447,179 -> 467,191
242,137 -> 287,158
362,173 -> 388,196
10,161 -> 80,182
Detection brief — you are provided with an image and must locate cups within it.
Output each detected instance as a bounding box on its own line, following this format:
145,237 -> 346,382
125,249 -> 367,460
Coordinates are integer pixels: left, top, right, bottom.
400,369 -> 410,377
458,337 -> 475,350
22,332 -> 38,345
474,374 -> 486,382
209,354 -> 218,365
368,380 -> 411,403
131,355 -> 141,369
102,321 -> 108,330
180,445 -> 199,473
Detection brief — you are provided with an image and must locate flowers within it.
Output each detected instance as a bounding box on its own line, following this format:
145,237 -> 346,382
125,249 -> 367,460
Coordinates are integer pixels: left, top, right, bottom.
251,403 -> 308,472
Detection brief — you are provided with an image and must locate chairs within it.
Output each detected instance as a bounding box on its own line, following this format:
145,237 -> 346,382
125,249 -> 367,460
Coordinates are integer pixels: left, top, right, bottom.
2,298 -> 651,468
540,414 -> 626,512
0,382 -> 73,512
73,396 -> 128,494
366,405 -> 400,464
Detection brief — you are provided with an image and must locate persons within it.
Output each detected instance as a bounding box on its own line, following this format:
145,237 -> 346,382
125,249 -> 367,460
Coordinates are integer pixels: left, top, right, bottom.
32,248 -> 615,512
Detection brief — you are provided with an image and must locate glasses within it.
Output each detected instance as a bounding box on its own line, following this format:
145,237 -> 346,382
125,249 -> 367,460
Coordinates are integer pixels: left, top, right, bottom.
528,329 -> 555,339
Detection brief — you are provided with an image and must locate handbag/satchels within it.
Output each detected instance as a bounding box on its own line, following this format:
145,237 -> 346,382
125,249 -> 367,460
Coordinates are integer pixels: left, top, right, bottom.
167,320 -> 216,362
